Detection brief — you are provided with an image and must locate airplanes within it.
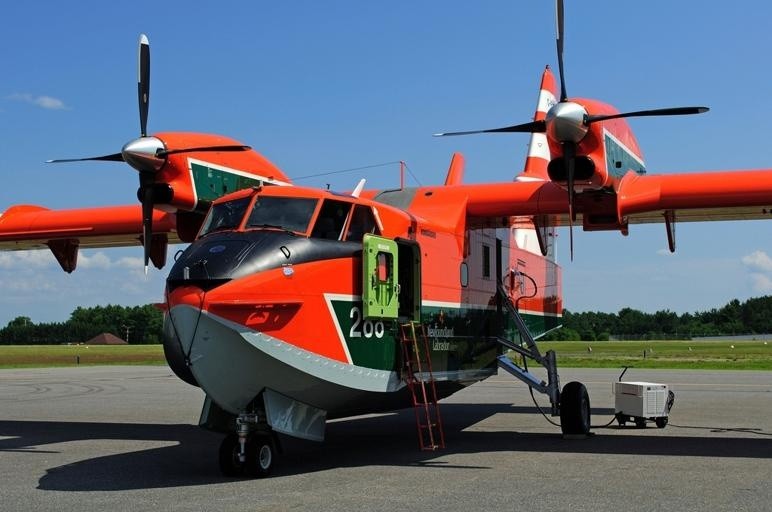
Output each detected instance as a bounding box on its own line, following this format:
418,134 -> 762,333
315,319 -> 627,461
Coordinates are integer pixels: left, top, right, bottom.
0,1 -> 772,479
